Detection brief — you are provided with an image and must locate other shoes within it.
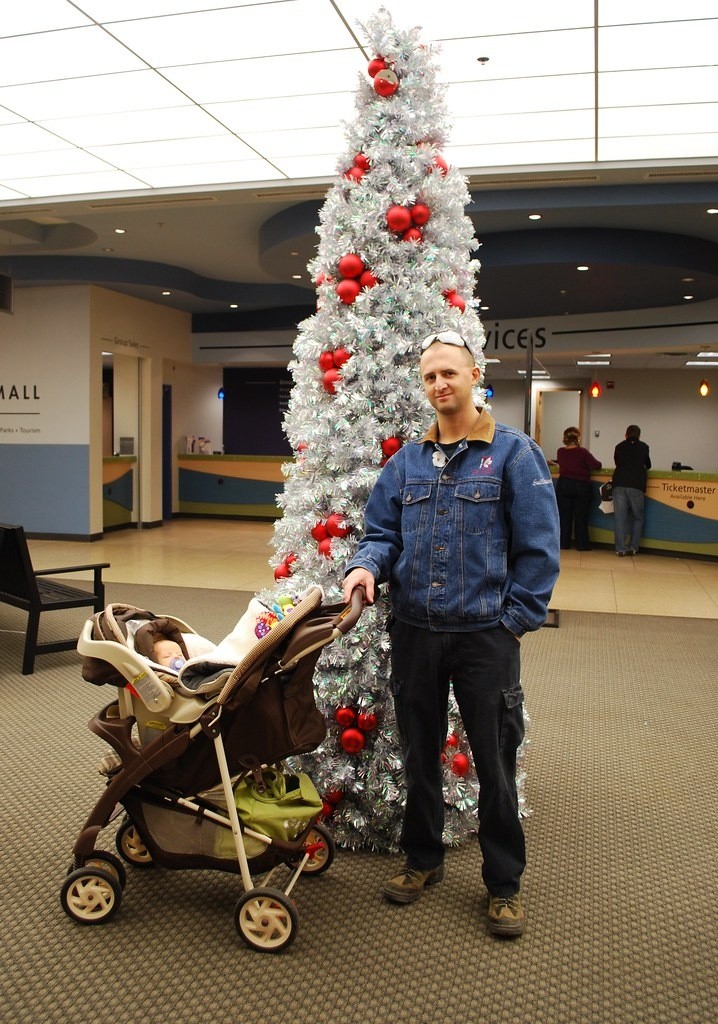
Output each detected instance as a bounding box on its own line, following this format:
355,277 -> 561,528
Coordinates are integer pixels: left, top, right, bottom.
628,548 -> 635,555
616,550 -> 626,556
577,545 -> 592,551
559,541 -> 569,549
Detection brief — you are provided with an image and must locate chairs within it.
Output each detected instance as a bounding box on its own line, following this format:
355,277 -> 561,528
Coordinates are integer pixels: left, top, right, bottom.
0,523 -> 111,676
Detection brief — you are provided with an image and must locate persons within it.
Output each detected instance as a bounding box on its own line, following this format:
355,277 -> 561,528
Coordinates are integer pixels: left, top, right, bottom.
612,424 -> 651,557
154,639 -> 187,672
556,427 -> 602,551
341,330 -> 561,936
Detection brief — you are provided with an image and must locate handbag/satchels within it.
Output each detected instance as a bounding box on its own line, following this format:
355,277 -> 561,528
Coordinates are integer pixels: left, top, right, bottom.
214,765 -> 324,859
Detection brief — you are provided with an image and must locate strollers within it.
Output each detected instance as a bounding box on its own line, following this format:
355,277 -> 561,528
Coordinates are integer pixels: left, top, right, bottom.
58,586 -> 381,954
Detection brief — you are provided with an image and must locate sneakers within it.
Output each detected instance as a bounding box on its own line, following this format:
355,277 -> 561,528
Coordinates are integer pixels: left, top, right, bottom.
488,892 -> 524,934
383,861 -> 445,903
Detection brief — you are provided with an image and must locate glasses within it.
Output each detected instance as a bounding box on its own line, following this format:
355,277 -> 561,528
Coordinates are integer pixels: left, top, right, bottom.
421,329 -> 472,356
